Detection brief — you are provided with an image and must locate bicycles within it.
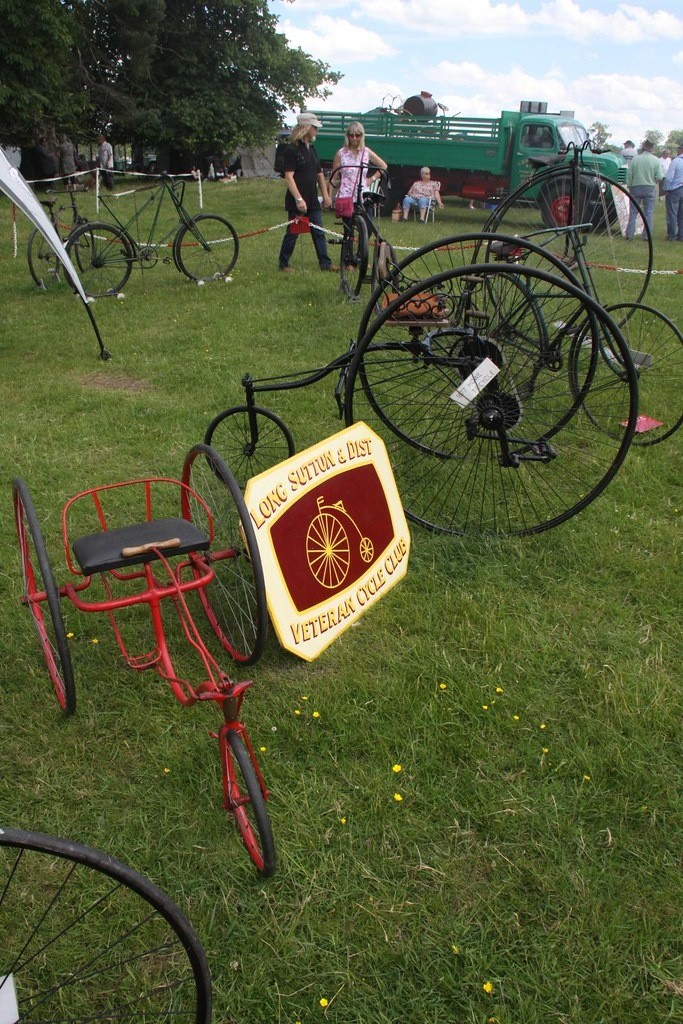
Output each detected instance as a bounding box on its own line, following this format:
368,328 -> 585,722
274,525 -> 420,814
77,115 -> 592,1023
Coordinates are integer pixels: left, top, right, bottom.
27,184 -> 101,288
63,170 -> 241,298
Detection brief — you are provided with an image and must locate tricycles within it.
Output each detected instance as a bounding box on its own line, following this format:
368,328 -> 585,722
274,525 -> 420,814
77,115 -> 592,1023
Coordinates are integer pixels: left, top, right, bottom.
8,433 -> 288,879
203,134 -> 683,540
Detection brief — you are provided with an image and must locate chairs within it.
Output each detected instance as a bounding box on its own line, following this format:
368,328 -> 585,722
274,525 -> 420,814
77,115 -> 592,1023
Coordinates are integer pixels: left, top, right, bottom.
411,181 -> 441,223
363,177 -> 384,218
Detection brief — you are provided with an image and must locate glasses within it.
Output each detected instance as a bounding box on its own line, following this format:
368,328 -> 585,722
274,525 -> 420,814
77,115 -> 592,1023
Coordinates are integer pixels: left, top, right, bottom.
348,133 -> 362,139
312,125 -> 318,130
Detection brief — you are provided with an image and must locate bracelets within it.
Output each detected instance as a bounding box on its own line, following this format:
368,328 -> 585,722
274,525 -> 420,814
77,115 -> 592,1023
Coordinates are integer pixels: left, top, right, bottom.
295,198 -> 303,204
369,178 -> 373,183
371,176 -> 376,181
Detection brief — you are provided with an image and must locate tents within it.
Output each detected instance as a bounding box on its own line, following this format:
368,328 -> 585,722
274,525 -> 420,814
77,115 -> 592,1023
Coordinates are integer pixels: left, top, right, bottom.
227,154 -> 274,178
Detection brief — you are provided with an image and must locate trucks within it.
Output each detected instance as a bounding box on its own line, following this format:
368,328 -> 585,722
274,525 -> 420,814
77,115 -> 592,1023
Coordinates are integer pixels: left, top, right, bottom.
294,99 -> 633,233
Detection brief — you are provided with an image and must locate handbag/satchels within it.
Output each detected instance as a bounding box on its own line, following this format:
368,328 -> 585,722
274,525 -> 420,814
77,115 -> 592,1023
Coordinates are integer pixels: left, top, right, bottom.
335,197 -> 354,218
392,202 -> 403,222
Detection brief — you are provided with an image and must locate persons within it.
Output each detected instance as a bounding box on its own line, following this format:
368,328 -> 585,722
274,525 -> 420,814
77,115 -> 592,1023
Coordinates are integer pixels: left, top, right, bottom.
658,147 -> 672,203
219,171 -> 237,184
398,166 -> 445,223
36,132 -> 78,191
191,165 -> 204,180
469,200 -> 476,211
662,142 -> 683,242
98,135 -> 115,191
626,140 -> 664,242
279,113 -> 340,273
327,121 -> 387,271
522,124 -> 549,148
619,141 -> 639,165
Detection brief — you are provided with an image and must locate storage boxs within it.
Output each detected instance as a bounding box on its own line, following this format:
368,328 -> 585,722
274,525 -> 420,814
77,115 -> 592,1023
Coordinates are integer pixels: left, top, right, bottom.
392,203 -> 403,221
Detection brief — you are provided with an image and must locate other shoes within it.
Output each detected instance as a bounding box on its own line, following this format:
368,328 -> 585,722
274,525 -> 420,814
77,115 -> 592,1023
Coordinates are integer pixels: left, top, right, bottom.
403,217 -> 408,221
321,265 -> 346,272
279,266 -> 297,273
345,265 -> 355,272
420,218 -> 424,223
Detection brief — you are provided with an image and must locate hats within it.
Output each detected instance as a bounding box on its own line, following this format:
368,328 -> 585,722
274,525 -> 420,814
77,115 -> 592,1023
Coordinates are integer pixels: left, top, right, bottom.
641,138 -> 654,152
677,142 -> 683,149
623,140 -> 635,148
297,113 -> 323,128
